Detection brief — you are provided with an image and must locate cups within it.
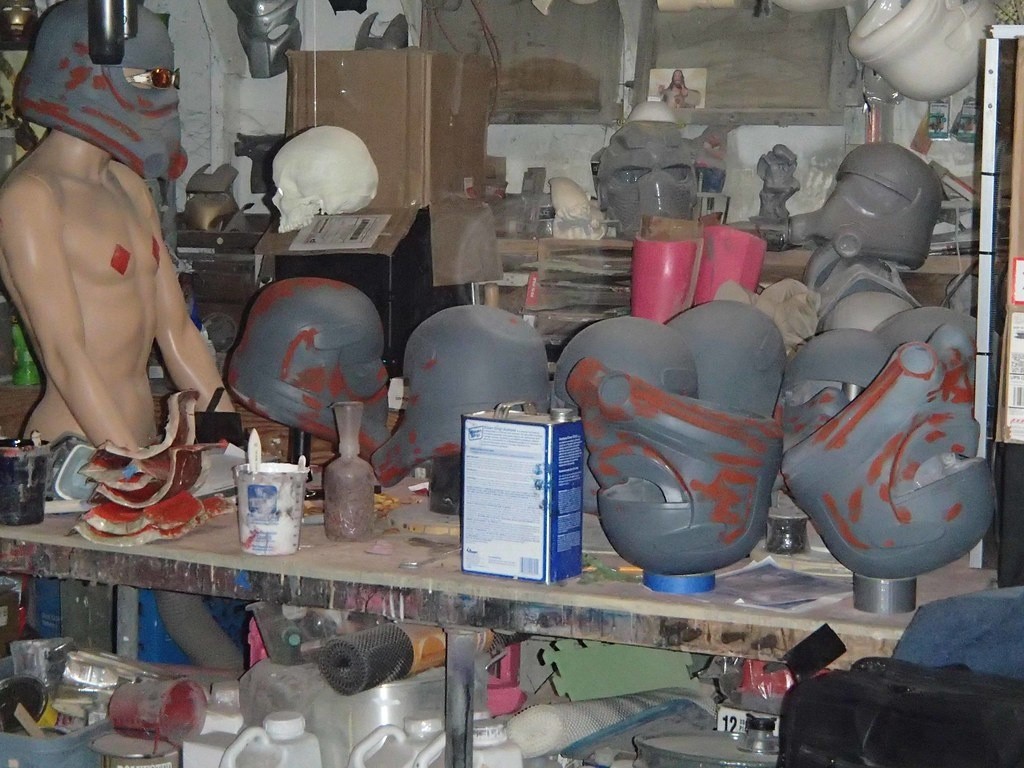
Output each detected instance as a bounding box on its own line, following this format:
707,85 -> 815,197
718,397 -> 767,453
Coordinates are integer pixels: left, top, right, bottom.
235,460 -> 310,556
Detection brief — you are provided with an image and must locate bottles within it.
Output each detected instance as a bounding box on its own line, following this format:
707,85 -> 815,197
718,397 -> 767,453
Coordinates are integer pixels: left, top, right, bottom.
324,443 -> 373,544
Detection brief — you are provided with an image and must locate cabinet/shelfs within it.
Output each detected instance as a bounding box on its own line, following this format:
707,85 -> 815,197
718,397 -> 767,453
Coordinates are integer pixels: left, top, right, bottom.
1,499 -> 1003,767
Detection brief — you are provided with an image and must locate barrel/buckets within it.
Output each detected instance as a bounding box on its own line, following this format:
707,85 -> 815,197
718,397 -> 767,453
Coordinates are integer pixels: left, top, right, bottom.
461,400 -> 584,585
217,710 -> 322,768
347,714 -> 523,768
110,679 -> 208,744
0,439 -> 49,526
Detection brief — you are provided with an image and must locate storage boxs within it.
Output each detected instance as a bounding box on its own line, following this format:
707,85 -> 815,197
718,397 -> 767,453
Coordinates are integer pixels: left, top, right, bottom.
253,45 -> 507,288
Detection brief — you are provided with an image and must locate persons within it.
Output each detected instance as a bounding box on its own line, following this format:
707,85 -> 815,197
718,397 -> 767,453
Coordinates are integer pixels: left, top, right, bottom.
0,0 -> 238,489
658,69 -> 702,109
